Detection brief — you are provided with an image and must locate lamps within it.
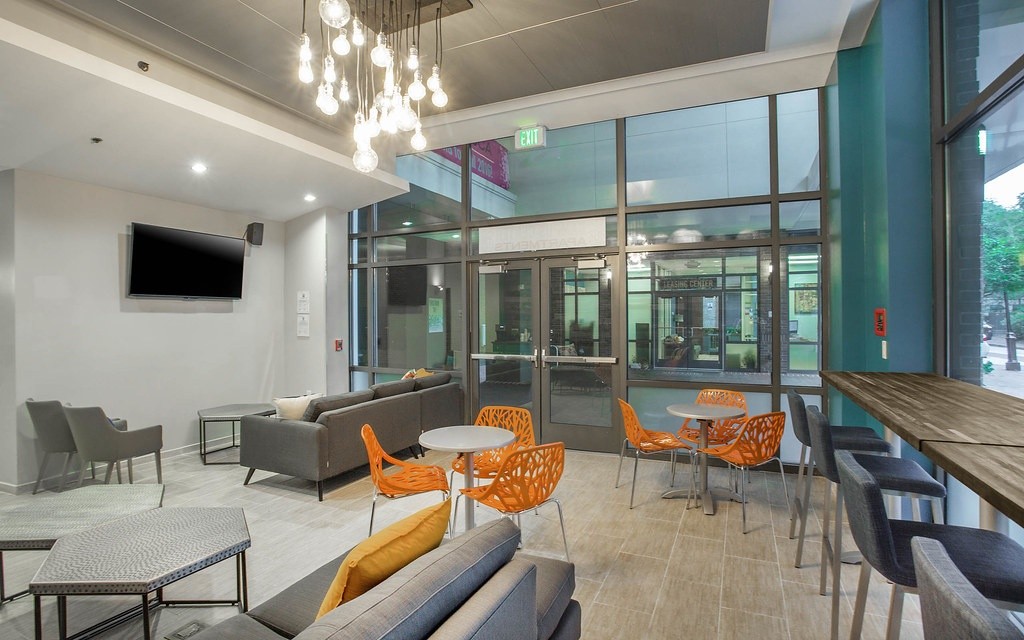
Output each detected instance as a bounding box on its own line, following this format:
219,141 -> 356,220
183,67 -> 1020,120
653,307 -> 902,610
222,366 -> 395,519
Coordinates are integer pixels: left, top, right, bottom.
298,0 -> 453,172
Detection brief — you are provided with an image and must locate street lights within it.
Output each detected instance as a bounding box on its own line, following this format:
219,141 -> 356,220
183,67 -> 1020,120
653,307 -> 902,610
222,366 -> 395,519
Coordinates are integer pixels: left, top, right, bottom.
1000,257 -> 1020,371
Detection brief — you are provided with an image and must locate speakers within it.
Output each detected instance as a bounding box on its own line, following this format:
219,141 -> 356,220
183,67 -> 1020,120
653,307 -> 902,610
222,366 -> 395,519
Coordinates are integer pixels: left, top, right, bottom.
247,223 -> 263,246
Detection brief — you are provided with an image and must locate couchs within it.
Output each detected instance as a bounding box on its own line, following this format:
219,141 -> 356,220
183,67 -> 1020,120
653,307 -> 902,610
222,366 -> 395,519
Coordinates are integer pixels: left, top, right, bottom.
186,536 -> 584,640
239,365 -> 463,502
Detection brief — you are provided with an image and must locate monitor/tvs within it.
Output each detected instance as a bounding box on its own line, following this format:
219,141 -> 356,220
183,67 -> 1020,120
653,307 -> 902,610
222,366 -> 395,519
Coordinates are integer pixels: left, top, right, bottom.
127,222 -> 245,300
387,264 -> 427,305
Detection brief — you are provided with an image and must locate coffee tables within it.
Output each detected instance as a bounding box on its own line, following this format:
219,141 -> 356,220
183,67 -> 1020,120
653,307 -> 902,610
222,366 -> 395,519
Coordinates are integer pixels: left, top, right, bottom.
27,500 -> 250,639
0,482 -> 167,602
196,402 -> 277,468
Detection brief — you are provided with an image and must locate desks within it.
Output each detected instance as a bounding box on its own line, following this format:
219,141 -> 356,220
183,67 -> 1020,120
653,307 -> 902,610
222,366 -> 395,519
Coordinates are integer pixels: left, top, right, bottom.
922,440 -> 1024,535
416,416 -> 515,536
819,368 -> 1023,530
660,402 -> 748,518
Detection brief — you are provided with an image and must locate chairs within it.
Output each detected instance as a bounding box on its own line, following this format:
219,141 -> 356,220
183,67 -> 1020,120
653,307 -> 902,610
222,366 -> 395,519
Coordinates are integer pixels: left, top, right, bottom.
664,389 -> 755,506
454,439 -> 571,565
831,445 -> 1024,638
614,397 -> 701,512
696,407 -> 791,534
781,387 -> 890,572
24,397 -> 129,495
805,405 -> 946,636
446,402 -> 542,524
912,535 -> 1024,640
63,402 -> 164,488
357,423 -> 452,543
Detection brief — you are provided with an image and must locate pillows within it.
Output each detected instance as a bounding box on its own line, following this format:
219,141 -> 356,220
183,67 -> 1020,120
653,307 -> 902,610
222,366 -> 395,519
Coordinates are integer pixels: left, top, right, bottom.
271,393 -> 325,423
398,369 -> 418,379
412,367 -> 436,378
312,496 -> 453,622
